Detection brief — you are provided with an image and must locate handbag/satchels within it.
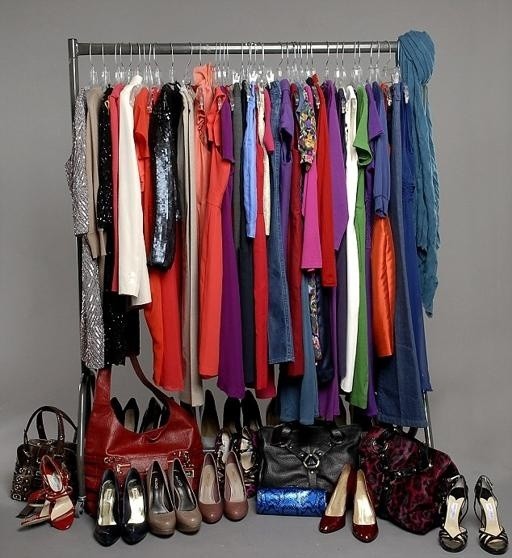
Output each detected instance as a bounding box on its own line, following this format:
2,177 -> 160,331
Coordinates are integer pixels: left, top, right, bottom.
84,400 -> 204,519
256,487 -> 330,517
359,425 -> 460,535
11,406 -> 78,504
257,420 -> 363,494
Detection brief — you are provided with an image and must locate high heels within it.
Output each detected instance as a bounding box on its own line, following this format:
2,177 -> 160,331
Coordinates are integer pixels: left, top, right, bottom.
223,451 -> 248,521
352,469 -> 378,543
474,475 -> 508,555
199,388 -> 220,450
332,397 -> 346,427
213,427 -> 232,490
139,397 -> 161,433
159,397 -> 174,427
198,453 -> 222,523
240,390 -> 263,432
146,460 -> 177,535
123,398 -> 139,432
223,397 -> 240,443
319,464 -> 356,533
94,469 -> 120,547
438,475 -> 468,552
233,426 -> 261,498
266,397 -> 279,425
121,468 -> 147,545
21,455 -> 75,530
167,458 -> 202,533
111,397 -> 122,425
